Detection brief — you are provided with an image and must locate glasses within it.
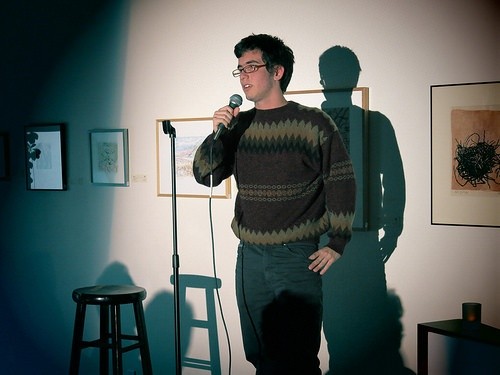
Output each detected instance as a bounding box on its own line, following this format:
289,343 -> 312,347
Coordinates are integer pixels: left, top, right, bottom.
232,64 -> 266,78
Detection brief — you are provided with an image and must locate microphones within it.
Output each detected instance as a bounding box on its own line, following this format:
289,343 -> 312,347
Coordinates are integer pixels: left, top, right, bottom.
211,94 -> 242,146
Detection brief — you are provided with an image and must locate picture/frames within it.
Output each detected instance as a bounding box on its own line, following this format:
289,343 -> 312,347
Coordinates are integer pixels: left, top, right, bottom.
23,124 -> 66,191
284,87 -> 369,232
88,129 -> 128,187
430,82 -> 500,228
156,117 -> 231,199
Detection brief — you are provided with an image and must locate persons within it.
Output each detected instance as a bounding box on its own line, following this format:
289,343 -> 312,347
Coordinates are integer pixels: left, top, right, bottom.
193,34 -> 357,375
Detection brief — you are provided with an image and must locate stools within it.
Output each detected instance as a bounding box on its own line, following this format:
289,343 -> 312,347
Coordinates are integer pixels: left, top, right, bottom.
70,285 -> 153,375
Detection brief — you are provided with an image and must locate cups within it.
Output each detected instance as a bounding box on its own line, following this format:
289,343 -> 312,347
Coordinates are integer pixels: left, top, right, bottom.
462,303 -> 481,327
